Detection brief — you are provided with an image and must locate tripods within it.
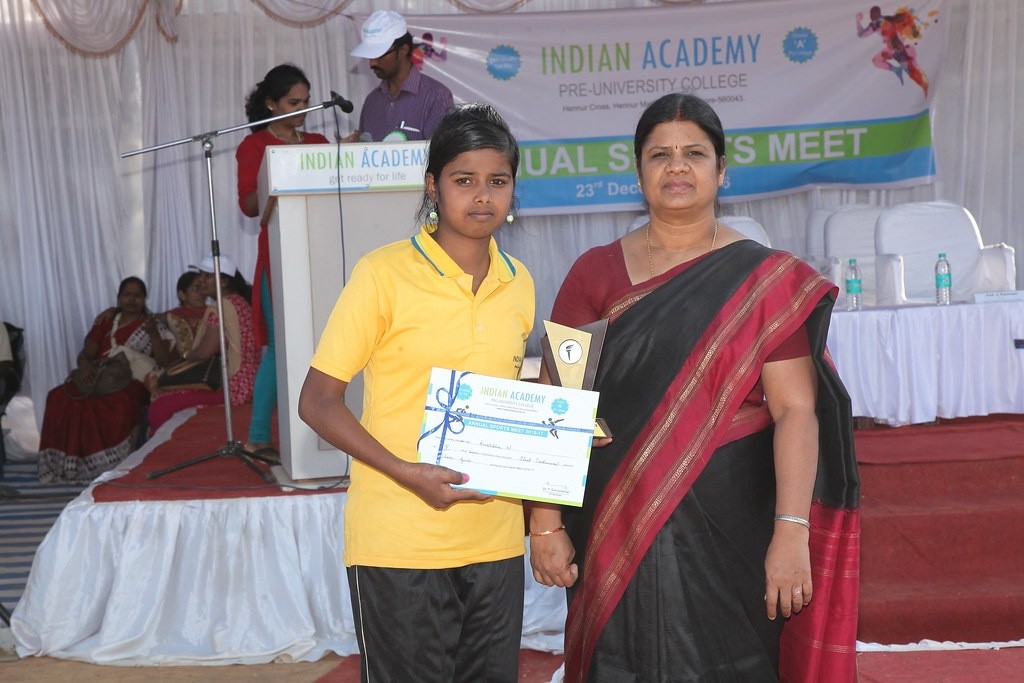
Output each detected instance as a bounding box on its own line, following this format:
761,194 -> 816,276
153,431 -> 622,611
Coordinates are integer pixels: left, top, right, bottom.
120,103 -> 331,484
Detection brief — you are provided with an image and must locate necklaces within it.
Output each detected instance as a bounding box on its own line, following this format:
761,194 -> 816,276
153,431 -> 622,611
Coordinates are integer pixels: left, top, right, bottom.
268,125 -> 301,142
646,221 -> 717,276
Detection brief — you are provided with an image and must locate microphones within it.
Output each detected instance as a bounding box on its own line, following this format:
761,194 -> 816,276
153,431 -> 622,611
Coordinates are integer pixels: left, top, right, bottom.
330,90 -> 354,113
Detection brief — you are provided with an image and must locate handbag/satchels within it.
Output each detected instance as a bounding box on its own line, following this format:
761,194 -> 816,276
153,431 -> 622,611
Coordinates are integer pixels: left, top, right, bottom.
64,352 -> 134,400
158,355 -> 221,392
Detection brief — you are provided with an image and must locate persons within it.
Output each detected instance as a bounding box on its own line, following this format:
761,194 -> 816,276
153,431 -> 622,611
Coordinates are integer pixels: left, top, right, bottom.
528,94 -> 861,683
235,64 -> 357,464
296,104 -> 610,683
36,276 -> 175,483
350,8 -> 454,141
135,252 -> 261,449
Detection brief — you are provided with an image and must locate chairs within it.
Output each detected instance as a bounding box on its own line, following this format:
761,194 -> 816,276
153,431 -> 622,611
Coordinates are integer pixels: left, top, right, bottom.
626,212 -> 772,250
803,201 -> 1016,307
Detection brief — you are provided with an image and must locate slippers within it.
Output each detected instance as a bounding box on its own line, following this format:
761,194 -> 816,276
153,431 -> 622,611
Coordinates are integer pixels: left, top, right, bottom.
241,443 -> 280,464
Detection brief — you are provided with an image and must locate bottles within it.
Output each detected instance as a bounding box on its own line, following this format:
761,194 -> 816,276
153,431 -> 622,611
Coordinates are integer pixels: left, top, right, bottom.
846,258 -> 862,312
935,253 -> 952,306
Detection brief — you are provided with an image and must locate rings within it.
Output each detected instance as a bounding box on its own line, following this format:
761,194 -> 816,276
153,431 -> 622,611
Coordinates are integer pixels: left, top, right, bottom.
792,588 -> 801,597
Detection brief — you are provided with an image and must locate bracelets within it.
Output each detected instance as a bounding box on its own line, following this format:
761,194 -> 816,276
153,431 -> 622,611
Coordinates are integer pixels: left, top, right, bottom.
529,524 -> 566,537
773,515 -> 810,528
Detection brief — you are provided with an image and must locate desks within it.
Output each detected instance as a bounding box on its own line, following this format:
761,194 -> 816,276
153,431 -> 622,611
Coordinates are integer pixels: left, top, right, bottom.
825,300 -> 1024,428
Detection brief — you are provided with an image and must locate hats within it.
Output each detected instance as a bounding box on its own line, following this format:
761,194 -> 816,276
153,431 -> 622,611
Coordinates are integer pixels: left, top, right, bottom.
188,254 -> 237,278
350,9 -> 407,59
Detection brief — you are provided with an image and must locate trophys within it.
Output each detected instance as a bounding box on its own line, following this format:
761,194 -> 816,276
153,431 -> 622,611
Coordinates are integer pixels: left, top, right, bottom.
540,318 -> 613,438
